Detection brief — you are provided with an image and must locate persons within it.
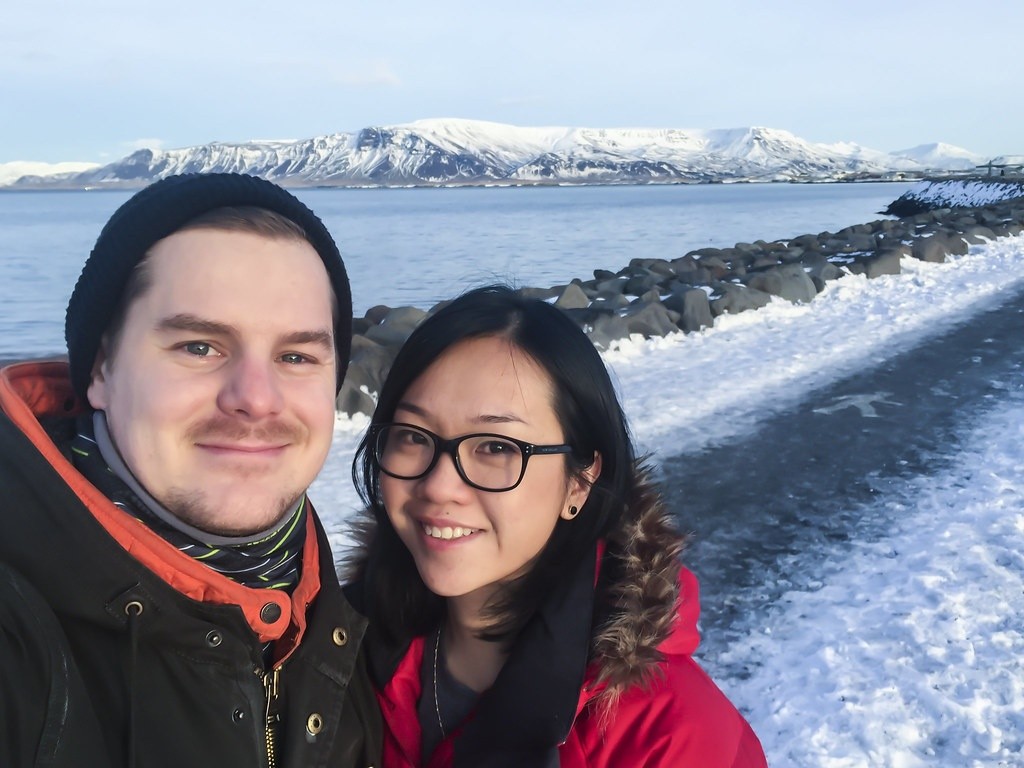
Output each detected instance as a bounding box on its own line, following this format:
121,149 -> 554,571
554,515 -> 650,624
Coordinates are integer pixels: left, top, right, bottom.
0,174 -> 383,768
340,284 -> 767,768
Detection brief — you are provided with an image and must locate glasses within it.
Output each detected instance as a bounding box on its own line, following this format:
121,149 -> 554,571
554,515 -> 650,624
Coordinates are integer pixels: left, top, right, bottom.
370,423 -> 573,492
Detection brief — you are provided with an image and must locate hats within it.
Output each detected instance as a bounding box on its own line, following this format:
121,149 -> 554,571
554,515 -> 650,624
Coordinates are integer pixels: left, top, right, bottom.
64,173 -> 354,420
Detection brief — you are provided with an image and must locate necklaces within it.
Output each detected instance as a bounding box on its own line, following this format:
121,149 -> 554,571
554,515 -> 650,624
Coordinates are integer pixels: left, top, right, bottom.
434,627 -> 447,740
